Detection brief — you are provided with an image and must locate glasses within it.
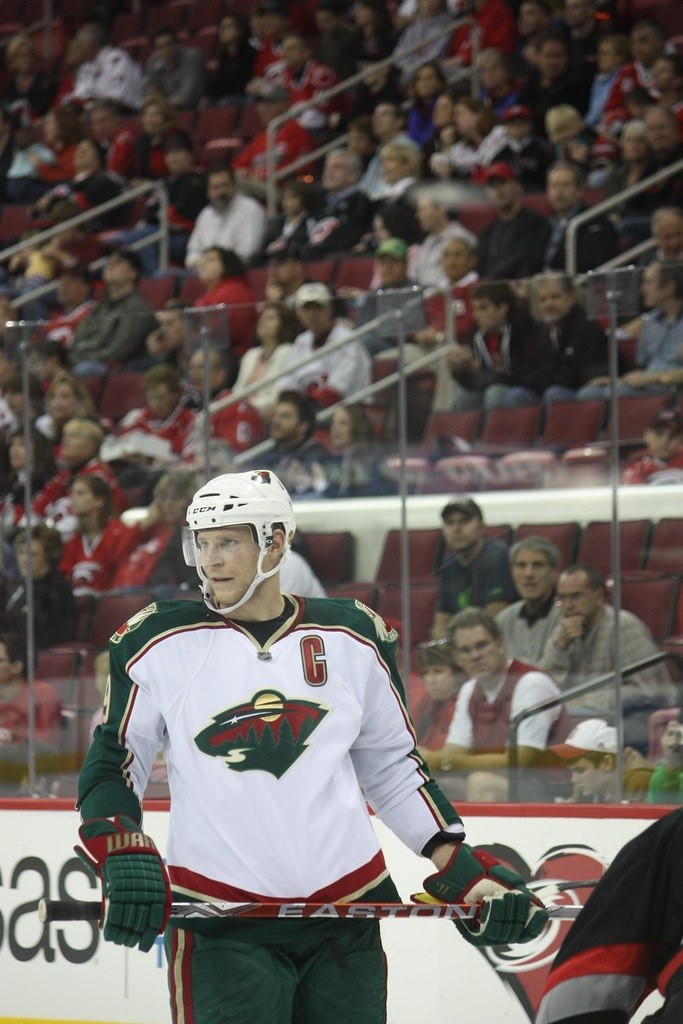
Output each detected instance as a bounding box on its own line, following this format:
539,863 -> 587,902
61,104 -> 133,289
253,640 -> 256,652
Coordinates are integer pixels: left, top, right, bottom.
558,591 -> 582,602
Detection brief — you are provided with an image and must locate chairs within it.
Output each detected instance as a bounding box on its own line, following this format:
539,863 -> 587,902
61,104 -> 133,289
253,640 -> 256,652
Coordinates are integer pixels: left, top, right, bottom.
0,0 -> 683,804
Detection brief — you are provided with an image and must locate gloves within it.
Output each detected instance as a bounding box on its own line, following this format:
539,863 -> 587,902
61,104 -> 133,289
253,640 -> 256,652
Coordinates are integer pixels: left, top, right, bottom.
73,813 -> 173,952
410,843 -> 549,947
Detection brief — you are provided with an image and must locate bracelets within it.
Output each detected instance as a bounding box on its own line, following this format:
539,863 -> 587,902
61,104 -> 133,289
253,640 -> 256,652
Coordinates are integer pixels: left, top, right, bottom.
45,190 -> 54,201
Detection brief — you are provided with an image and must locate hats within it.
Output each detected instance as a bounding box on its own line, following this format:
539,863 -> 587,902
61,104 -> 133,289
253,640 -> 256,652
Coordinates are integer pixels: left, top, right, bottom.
378,240 -> 407,260
548,718 -> 618,759
504,105 -> 529,121
442,498 -> 482,525
481,162 -> 513,181
649,405 -> 683,433
247,77 -> 289,101
297,282 -> 330,307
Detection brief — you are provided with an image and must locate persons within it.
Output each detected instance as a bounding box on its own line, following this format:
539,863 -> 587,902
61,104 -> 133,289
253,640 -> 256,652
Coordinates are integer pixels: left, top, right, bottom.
1,0 -> 682,804
76,470 -> 547,1024
534,806 -> 683,1023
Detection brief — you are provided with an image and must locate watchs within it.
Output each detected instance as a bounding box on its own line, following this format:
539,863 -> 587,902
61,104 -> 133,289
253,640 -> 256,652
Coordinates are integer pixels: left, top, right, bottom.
441,749 -> 453,772
658,372 -> 670,385
435,331 -> 445,343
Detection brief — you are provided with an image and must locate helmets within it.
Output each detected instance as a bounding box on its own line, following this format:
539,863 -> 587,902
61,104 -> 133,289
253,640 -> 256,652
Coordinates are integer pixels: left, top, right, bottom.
186,470 -> 295,554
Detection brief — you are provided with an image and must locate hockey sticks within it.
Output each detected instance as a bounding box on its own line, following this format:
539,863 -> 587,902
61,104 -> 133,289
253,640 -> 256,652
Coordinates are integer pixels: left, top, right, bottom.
41,902 -> 582,924
